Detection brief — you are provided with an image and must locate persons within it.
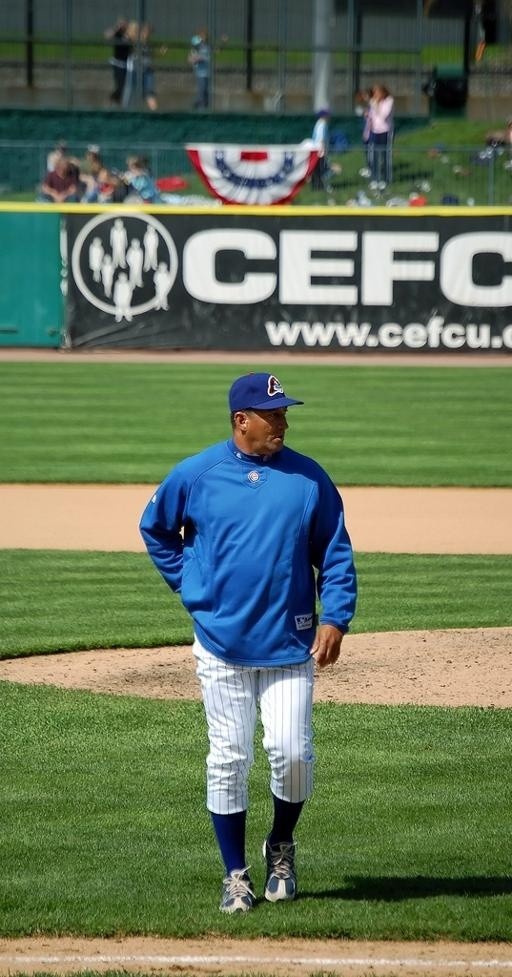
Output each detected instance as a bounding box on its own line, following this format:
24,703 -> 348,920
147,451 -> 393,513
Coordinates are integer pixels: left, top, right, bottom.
34,140 -> 163,204
188,23 -> 229,111
102,16 -> 168,111
312,108 -> 332,191
354,82 -> 396,192
139,372 -> 356,915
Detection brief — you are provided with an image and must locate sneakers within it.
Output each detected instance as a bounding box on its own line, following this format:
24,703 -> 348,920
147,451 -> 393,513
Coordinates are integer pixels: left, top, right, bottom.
220,867 -> 254,913
262,833 -> 297,902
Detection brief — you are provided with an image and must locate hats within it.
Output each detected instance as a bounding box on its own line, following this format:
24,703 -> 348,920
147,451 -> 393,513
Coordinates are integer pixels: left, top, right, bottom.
229,372 -> 304,412
